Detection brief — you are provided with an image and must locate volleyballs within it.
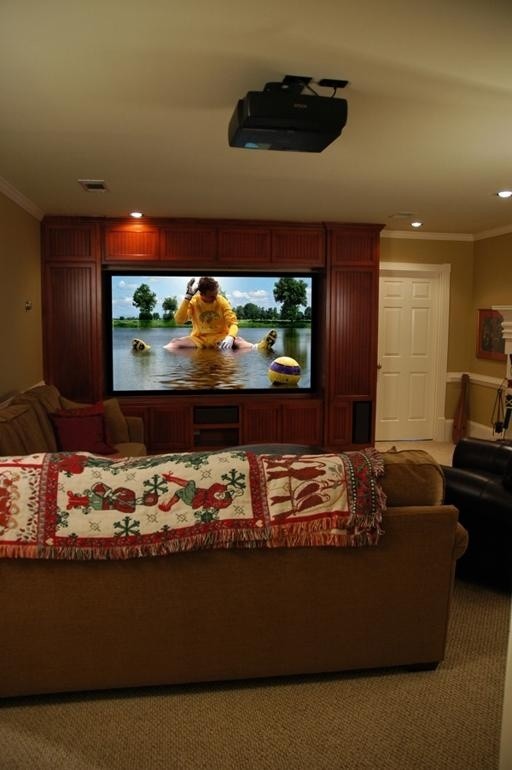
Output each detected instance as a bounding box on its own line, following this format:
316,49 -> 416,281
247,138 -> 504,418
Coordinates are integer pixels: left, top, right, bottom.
269,356 -> 300,386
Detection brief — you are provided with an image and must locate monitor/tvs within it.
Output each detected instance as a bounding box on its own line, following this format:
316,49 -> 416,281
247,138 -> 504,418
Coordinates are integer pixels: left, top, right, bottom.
106,270 -> 317,396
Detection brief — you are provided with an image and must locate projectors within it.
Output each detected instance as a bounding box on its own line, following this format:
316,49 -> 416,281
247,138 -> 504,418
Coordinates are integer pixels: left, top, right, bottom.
228,91 -> 347,153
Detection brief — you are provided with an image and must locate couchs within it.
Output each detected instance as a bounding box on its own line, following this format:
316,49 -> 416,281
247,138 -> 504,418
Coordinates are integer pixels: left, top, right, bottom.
1,382 -> 468,703
2,383 -> 147,462
2,446 -> 468,704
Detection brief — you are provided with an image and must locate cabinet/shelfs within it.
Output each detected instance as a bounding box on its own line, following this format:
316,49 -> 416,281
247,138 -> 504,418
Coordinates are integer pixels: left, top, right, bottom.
118,395 -> 325,452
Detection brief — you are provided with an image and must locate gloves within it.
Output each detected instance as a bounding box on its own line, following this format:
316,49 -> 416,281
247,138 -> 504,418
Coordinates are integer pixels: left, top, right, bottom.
218,335 -> 238,350
184,276 -> 199,301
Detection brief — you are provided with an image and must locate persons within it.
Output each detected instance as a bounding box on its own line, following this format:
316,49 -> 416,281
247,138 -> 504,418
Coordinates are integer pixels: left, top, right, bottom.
132,277 -> 277,354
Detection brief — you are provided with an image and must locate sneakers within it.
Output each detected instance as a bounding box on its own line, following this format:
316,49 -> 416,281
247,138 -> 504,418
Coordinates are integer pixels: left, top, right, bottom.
131,336 -> 154,354
257,328 -> 278,353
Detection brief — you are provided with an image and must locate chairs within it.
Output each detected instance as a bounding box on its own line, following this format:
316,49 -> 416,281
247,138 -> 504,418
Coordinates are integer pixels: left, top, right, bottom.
436,434 -> 512,596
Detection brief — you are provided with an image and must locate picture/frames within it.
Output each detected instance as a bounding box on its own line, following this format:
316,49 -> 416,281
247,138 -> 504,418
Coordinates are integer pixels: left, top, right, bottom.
473,308 -> 506,361
